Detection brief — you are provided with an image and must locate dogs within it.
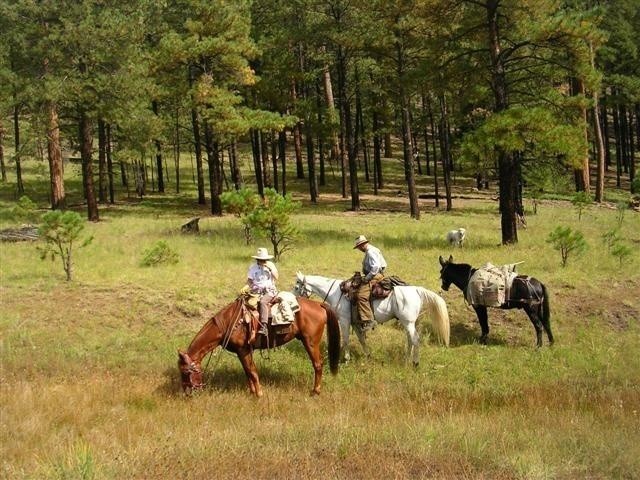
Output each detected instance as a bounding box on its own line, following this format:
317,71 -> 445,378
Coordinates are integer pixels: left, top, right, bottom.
444,228 -> 465,249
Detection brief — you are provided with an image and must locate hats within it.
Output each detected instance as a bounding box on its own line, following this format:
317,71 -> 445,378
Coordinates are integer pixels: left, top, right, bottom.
353,235 -> 370,249
250,247 -> 274,261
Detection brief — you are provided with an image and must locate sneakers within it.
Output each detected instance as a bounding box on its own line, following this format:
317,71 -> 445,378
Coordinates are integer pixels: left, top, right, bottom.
258,324 -> 269,336
361,320 -> 377,331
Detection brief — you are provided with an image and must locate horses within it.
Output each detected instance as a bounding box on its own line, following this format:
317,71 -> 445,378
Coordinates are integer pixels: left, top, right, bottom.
439,255 -> 554,352
292,271 -> 451,373
178,294 -> 340,406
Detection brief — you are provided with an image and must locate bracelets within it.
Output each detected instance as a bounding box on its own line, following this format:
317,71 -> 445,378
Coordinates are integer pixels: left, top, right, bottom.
268,266 -> 273,272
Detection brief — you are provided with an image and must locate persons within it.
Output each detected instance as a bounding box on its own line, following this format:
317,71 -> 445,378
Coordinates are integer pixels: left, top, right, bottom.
351,234 -> 387,331
238,247 -> 279,337
